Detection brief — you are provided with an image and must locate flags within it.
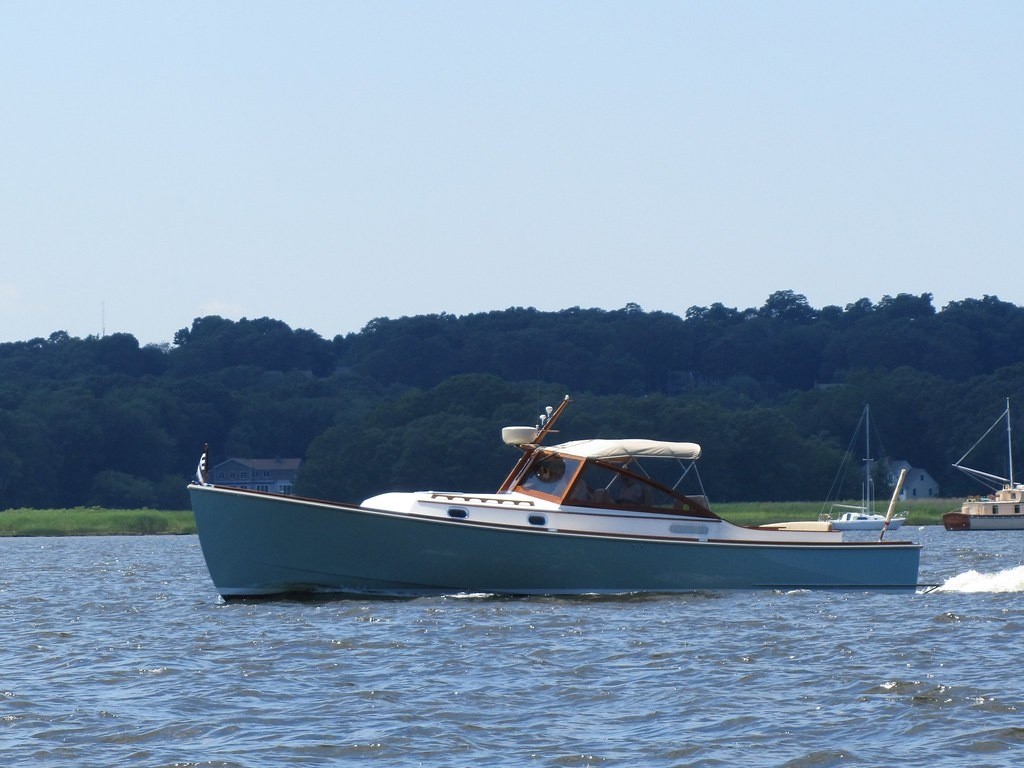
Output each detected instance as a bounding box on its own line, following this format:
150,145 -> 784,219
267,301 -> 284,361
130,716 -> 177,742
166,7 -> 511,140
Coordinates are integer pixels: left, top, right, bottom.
195,450 -> 214,487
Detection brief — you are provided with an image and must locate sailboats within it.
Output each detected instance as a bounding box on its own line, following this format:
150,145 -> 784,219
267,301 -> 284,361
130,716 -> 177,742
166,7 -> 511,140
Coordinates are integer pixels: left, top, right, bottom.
756,404 -> 909,531
943,398 -> 1024,528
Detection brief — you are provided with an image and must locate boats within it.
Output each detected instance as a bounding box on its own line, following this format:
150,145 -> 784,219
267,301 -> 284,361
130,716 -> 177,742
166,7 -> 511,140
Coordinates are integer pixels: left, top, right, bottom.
185,394 -> 923,601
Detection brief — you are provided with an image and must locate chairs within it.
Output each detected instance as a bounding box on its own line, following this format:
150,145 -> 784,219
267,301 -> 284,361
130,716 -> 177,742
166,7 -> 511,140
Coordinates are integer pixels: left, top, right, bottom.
675,493 -> 710,516
594,489 -> 615,504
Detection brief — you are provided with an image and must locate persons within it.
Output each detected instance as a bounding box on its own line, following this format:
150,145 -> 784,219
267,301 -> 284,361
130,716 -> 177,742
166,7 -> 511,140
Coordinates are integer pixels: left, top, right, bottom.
618,469 -> 643,504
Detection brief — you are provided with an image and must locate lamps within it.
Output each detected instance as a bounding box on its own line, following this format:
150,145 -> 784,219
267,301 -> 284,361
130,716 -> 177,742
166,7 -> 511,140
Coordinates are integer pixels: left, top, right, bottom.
546,405 -> 553,422
540,414 -> 546,428
